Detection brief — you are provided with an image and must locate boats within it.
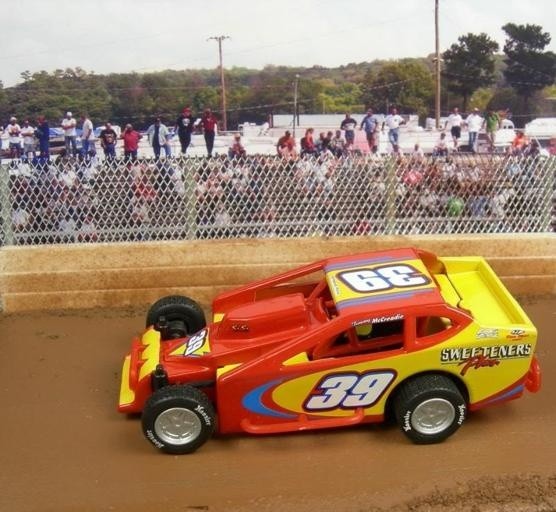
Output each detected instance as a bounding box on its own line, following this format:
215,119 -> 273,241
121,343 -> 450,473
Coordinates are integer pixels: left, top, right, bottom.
45,128 -> 99,147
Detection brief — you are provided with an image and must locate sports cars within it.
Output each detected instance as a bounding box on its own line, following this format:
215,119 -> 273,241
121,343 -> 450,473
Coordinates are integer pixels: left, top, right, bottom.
116,246 -> 543,455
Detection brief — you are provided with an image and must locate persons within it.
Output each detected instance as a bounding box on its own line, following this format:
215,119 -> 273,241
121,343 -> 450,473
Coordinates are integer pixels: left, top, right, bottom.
403,107 -> 556,233
275,106 -> 405,234
331,314 -> 372,342
218,120 -> 276,238
1,105 -> 219,244
